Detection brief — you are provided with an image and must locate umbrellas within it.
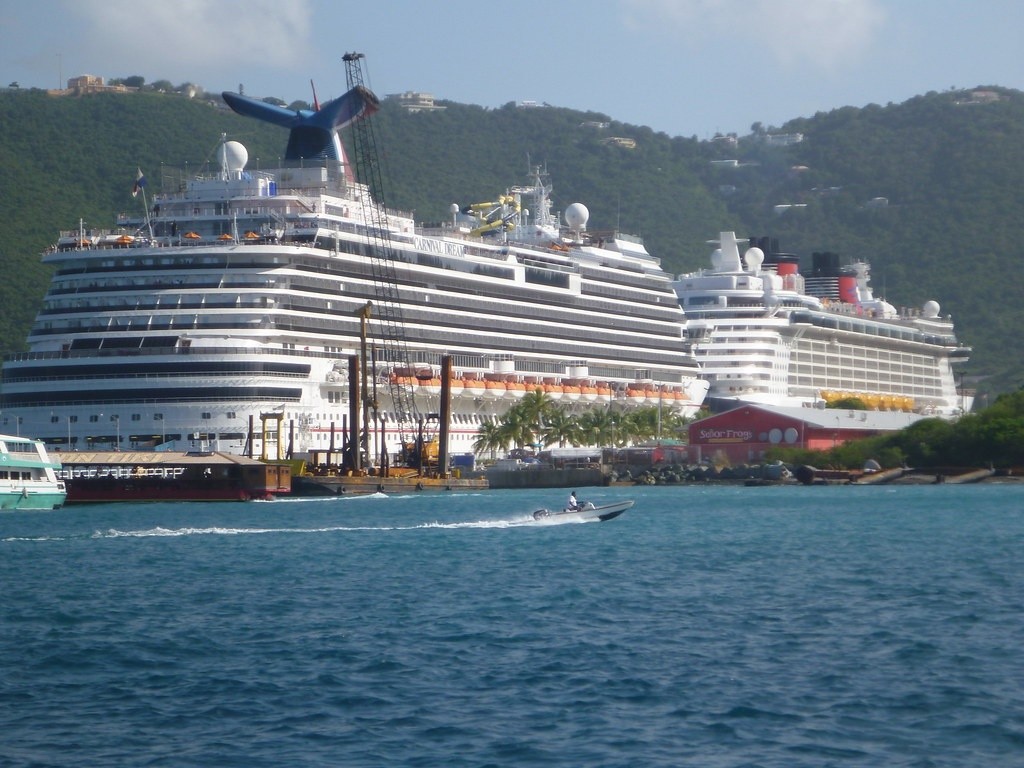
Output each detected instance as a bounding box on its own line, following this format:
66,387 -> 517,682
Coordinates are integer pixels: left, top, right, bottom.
77,231 -> 261,244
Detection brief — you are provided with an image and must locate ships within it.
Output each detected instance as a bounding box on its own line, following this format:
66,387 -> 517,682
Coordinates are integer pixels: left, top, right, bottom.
0,87 -> 713,466
672,230 -> 977,414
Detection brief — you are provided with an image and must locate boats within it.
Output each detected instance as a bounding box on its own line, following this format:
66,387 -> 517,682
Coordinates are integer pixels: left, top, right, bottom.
532,500 -> 637,523
290,301 -> 491,495
0,434 -> 68,513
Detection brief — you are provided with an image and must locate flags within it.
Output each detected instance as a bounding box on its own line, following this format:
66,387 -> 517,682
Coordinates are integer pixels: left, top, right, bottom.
133,168 -> 145,197
273,404 -> 286,410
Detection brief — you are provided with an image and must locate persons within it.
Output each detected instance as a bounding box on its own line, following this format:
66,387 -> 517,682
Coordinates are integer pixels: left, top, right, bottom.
569,491 -> 578,508
312,204 -> 316,212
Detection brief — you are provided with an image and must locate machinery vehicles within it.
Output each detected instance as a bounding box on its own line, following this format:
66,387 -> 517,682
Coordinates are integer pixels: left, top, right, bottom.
341,52 -> 454,470
